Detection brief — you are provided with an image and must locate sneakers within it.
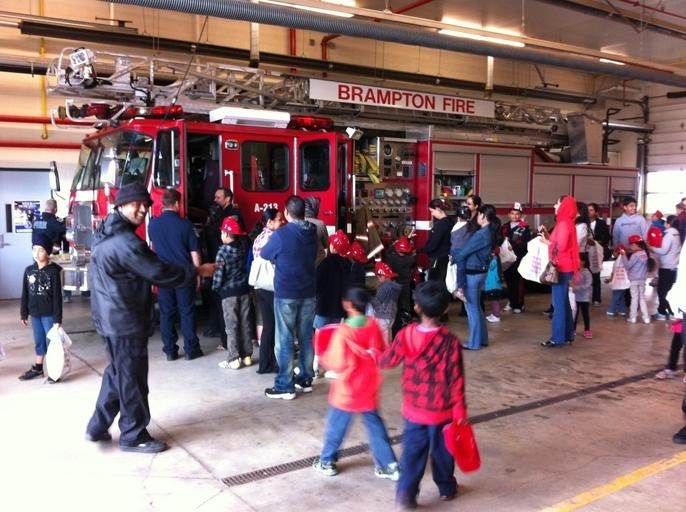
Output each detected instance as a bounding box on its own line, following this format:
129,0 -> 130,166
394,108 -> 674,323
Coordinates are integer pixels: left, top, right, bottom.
118,436 -> 168,453
263,386 -> 297,400
373,462 -> 400,480
293,374 -> 312,394
313,457 -> 339,477
607,309 -> 676,324
242,355 -> 252,366
456,291 -> 468,303
17,364 -> 44,382
440,476 -> 459,501
85,425 -> 113,441
656,369 -> 674,380
460,299 -> 527,350
217,357 -> 242,370
540,300 -> 602,348
394,491 -> 419,511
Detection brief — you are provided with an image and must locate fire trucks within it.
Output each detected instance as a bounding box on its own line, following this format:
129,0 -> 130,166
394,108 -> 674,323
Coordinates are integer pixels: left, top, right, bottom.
44,45 -> 642,327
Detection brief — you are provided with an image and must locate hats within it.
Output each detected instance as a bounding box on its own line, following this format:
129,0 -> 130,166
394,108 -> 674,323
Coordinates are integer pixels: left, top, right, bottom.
350,241 -> 368,263
113,184 -> 155,206
373,261 -> 399,278
415,253 -> 431,269
508,202 -> 524,213
328,228 -> 351,256
444,421 -> 481,472
219,217 -> 248,236
627,234 -> 640,243
31,233 -> 53,255
395,235 -> 412,253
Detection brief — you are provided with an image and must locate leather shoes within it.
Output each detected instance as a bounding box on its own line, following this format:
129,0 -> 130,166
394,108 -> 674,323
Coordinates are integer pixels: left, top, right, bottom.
167,349 -> 178,360
185,348 -> 202,360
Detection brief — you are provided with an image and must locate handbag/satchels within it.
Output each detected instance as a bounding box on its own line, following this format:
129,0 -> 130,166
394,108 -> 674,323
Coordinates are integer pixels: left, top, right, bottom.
516,233 -> 550,285
248,232 -> 275,292
539,248 -> 561,286
585,241 -> 604,274
499,236 -> 518,272
611,254 -> 630,291
43,316 -> 75,382
445,257 -> 458,294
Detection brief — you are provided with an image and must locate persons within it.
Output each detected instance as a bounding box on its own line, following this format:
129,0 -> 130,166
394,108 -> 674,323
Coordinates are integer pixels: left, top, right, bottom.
31,199 -> 64,256
673,373 -> 686,445
212,214 -> 254,370
61,216 -> 90,303
84,184 -> 222,453
259,196 -> 319,400
146,188 -> 203,360
312,284 -> 400,480
197,187 -> 257,349
301,192 -> 686,382
17,233 -> 62,383
368,280 -> 469,512
248,209 -> 286,374
